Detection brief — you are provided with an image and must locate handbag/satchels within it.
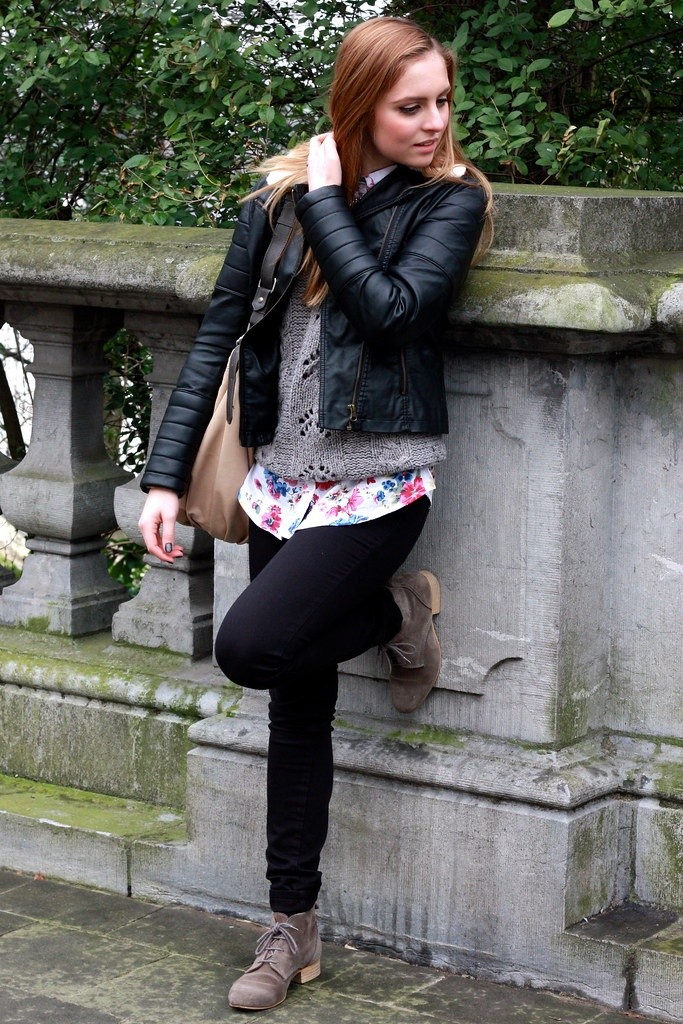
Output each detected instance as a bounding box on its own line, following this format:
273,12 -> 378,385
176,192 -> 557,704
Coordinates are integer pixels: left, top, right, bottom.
174,183 -> 306,547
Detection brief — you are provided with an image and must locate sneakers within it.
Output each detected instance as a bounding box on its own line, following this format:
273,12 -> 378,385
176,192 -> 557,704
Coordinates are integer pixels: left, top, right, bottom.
226,908 -> 324,1010
376,571 -> 441,715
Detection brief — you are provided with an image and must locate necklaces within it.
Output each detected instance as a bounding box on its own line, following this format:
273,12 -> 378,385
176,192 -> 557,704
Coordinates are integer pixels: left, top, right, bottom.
348,192 -> 366,209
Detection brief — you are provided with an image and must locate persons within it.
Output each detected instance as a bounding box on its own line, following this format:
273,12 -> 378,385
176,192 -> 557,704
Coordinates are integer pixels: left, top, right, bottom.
139,17 -> 495,1010
